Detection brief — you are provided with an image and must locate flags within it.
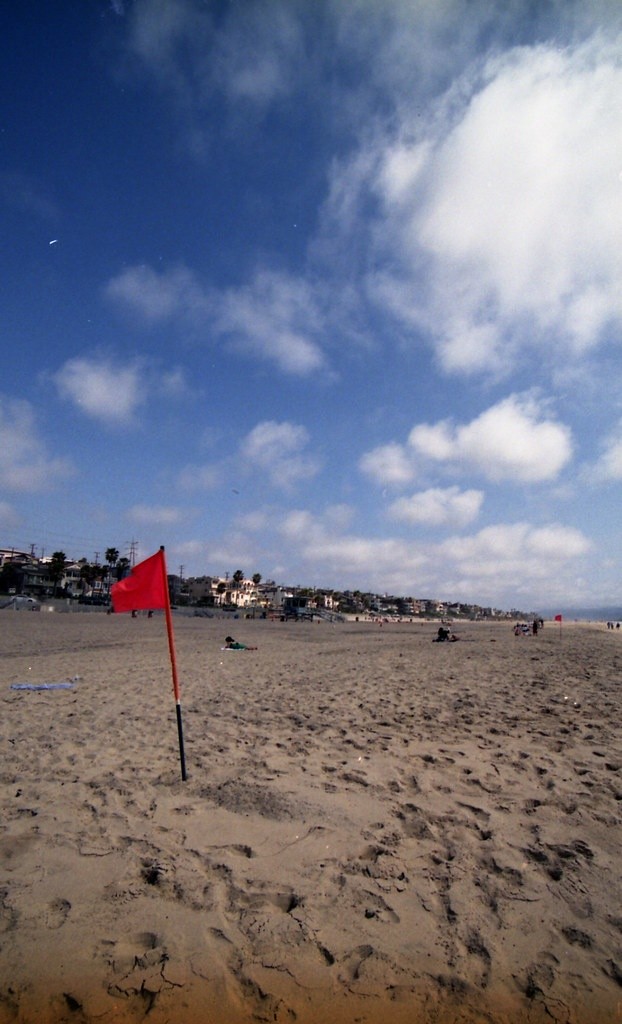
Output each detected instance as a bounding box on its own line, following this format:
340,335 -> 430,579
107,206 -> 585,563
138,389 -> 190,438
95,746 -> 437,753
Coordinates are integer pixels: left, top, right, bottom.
111,549 -> 170,613
555,615 -> 561,622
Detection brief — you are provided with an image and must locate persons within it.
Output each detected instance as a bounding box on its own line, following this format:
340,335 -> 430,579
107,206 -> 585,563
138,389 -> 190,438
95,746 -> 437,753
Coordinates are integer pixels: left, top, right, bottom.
607,622 -> 620,629
107,558 -> 138,619
225,636 -> 258,650
514,618 -> 544,636
148,609 -> 154,618
438,627 -> 450,642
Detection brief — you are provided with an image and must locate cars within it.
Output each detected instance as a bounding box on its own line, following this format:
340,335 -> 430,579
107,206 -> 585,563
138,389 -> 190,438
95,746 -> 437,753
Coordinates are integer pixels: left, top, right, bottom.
10,594 -> 37,606
282,605 -> 314,622
78,591 -> 110,607
